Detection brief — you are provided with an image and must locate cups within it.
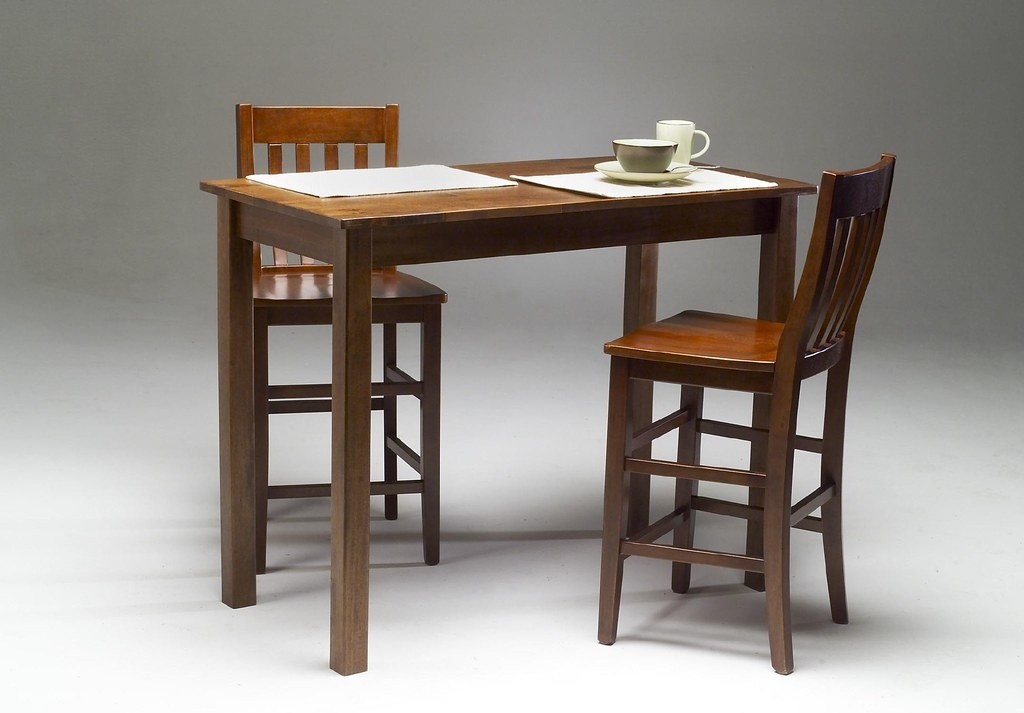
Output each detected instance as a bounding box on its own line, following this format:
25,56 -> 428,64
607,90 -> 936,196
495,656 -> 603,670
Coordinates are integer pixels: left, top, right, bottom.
656,120 -> 710,171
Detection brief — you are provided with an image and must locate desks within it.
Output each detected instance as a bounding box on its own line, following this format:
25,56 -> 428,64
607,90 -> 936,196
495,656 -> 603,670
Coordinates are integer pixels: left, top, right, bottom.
200,155 -> 817,679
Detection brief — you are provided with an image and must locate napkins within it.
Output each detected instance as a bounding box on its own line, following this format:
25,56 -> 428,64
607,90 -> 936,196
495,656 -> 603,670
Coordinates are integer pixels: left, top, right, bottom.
246,163 -> 520,198
508,167 -> 781,200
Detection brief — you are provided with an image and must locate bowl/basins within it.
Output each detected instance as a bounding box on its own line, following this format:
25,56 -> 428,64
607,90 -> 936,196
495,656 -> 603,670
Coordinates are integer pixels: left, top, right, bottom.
612,139 -> 678,173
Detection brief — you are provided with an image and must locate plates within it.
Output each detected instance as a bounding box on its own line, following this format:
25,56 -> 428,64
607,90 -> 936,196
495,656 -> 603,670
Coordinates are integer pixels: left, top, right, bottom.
593,161 -> 697,184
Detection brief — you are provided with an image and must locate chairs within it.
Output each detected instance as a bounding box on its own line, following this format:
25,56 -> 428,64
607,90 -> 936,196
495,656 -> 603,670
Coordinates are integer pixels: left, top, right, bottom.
597,152 -> 897,674
235,100 -> 449,573
